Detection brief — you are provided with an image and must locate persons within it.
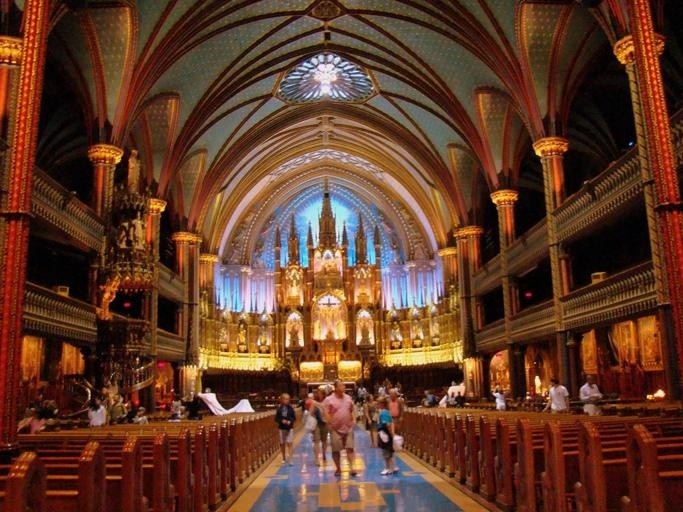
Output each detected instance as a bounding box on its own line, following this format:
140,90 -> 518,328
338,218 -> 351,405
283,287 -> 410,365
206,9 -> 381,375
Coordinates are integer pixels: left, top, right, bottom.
578,375 -> 603,416
411,319 -> 420,339
540,376 -> 570,415
431,317 -> 440,338
260,326 -> 267,345
391,321 -> 399,341
325,328 -> 334,340
296,376 -> 406,478
492,385 -> 506,411
274,391 -> 297,467
421,389 -> 464,407
288,325 -> 300,348
237,329 -> 245,344
358,322 -> 371,346
15,376 -> 205,435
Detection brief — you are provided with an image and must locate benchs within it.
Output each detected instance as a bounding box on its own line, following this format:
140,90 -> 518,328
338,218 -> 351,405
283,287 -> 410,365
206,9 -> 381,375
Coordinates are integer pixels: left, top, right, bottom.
401,407 -> 683,512
1,411 -> 278,511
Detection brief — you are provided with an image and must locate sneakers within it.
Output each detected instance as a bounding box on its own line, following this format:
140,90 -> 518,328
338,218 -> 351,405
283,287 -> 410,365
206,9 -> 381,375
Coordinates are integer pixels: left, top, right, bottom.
281,460 -> 294,466
381,468 -> 399,475
335,469 -> 340,475
314,453 -> 325,465
350,469 -> 356,475
